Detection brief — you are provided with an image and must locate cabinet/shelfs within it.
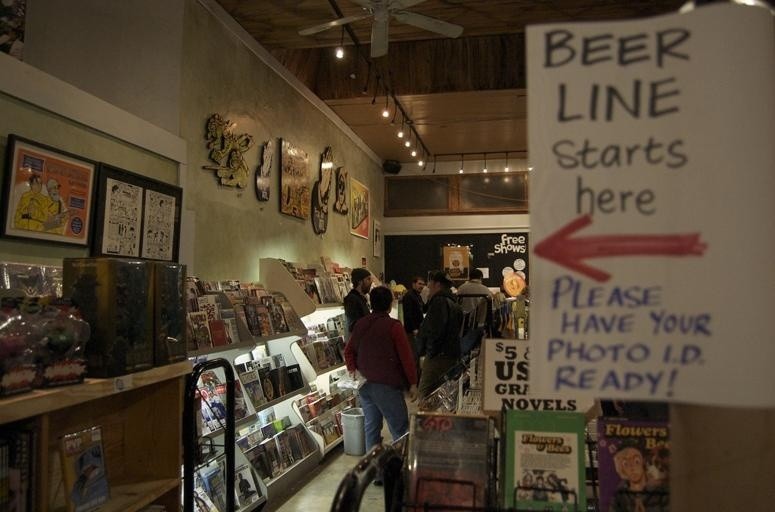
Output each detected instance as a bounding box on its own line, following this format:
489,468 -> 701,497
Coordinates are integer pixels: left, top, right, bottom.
0,253 -> 380,512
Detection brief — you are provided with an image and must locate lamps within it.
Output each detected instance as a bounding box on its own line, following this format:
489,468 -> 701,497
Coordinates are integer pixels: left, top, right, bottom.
333,25 -> 528,175
374,7 -> 388,22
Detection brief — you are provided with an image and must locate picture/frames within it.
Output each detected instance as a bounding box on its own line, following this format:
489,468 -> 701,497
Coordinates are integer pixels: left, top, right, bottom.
0,134 -> 99,250
58,425 -> 111,512
91,161 -> 182,262
349,178 -> 371,240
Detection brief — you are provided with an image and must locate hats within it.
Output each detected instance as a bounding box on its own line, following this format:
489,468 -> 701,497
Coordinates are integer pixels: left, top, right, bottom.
351,268 -> 371,281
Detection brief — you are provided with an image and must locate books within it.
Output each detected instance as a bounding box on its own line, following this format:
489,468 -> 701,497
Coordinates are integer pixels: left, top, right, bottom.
1,421 -> 114,512
404,397 -> 672,512
183,255 -> 357,512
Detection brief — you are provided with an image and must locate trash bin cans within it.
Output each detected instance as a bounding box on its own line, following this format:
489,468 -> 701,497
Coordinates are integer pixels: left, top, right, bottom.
341,407 -> 366,456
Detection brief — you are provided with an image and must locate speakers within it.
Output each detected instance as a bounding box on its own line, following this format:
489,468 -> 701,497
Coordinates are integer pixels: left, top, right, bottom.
383,160 -> 401,174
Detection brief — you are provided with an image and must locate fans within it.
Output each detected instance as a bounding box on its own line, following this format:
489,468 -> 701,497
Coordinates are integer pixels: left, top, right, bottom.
297,0 -> 465,58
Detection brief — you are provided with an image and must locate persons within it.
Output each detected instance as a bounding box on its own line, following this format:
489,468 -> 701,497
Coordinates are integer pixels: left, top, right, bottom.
401,275 -> 425,392
413,271 -> 465,404
341,285 -> 421,487
342,267 -> 373,343
457,268 -> 493,313
